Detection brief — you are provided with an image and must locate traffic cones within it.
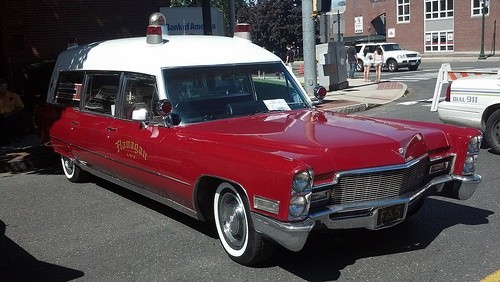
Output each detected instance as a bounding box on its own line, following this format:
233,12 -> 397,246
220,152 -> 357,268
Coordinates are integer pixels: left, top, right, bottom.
299,64 -> 304,74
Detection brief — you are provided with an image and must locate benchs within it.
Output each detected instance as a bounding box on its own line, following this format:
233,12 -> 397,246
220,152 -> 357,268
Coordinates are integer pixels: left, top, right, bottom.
129,90 -> 254,121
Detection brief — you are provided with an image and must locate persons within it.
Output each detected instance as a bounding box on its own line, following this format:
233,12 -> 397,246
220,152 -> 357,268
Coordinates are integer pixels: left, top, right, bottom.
257,70 -> 266,79
361,46 -> 372,82
213,69 -> 257,119
285,44 -> 294,72
0,79 -> 24,146
372,48 -> 384,83
346,41 -> 358,79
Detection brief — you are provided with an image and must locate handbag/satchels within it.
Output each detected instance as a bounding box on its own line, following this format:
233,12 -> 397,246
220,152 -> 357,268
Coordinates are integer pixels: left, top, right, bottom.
374,63 -> 379,67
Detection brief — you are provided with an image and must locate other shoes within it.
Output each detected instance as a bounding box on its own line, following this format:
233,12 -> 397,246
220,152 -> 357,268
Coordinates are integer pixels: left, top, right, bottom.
37,168 -> 61,174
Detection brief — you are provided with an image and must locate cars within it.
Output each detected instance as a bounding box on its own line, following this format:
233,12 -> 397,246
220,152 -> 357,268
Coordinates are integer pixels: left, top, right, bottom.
430,62 -> 500,154
33,11 -> 487,268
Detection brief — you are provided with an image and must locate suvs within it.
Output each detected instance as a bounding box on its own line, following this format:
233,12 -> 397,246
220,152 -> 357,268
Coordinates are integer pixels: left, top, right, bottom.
354,42 -> 421,72
